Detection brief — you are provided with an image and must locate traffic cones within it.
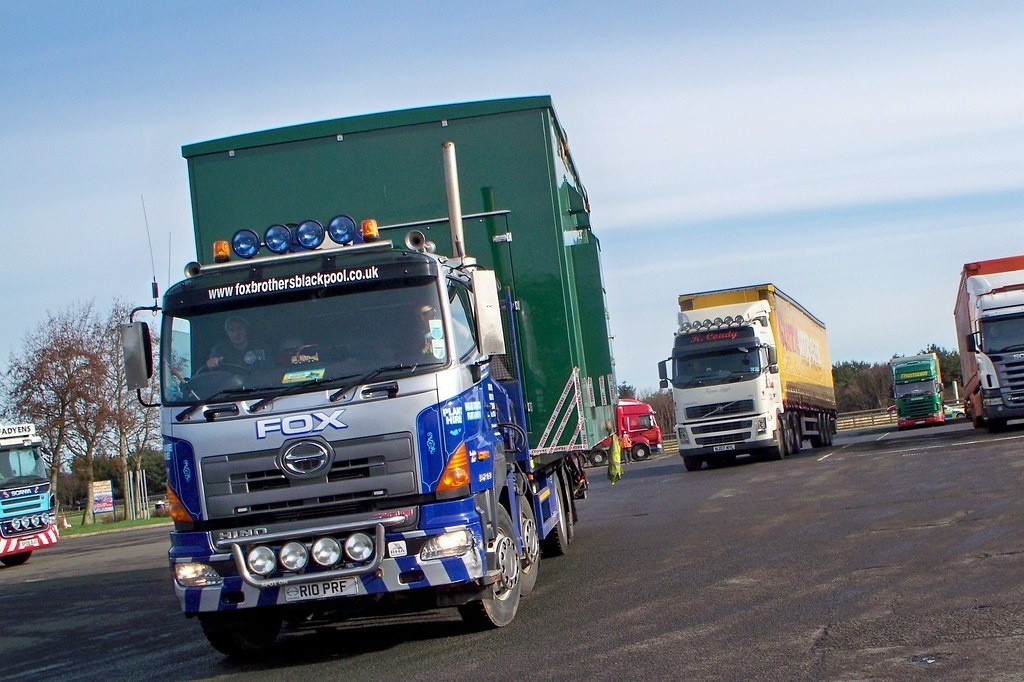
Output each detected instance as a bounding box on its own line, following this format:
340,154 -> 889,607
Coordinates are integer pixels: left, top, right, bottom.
61,514 -> 68,529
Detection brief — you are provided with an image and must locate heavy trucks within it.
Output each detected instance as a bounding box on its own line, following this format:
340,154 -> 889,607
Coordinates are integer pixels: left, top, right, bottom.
656,283 -> 839,471
113,95 -> 622,658
576,397 -> 662,468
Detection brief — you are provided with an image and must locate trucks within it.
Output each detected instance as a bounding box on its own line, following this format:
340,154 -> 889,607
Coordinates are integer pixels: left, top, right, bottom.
953,255 -> 1023,434
1,423 -> 72,568
890,351 -> 946,429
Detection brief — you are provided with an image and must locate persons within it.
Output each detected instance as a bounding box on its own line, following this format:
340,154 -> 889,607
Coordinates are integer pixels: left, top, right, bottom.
207,315 -> 265,375
391,299 -> 469,366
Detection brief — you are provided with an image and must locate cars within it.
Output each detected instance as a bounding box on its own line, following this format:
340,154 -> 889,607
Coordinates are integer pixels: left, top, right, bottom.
943,404 -> 965,421
887,404 -> 897,414
